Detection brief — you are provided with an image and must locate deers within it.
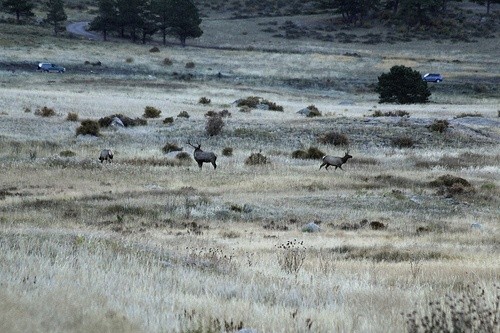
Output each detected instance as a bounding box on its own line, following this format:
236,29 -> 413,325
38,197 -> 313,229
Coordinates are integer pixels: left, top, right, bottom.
185,140 -> 217,170
319,149 -> 352,173
99,149 -> 113,164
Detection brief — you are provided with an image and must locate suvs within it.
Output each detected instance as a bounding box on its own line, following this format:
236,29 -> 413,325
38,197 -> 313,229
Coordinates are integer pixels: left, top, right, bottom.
422,72 -> 444,83
38,63 -> 66,73
